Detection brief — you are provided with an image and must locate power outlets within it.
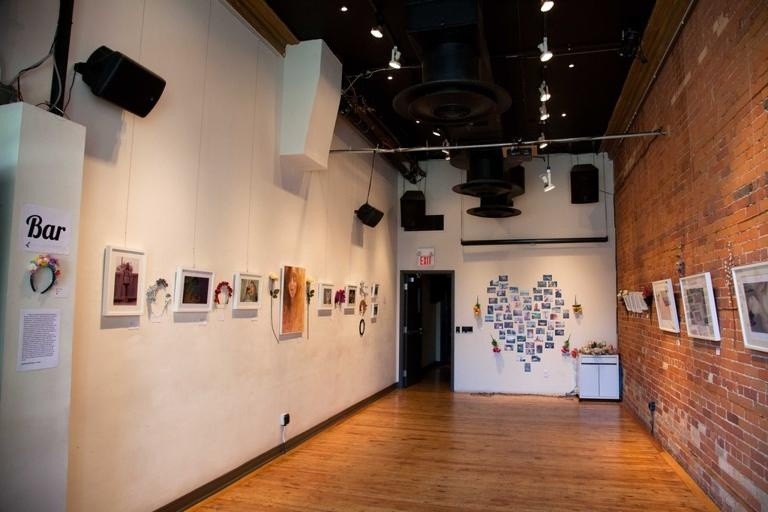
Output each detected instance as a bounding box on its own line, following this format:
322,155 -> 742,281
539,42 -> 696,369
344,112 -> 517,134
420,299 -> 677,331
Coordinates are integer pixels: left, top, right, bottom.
280,412 -> 289,426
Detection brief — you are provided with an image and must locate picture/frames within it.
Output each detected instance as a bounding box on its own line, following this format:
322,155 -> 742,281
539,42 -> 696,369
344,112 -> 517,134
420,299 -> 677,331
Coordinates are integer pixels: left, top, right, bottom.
372,283 -> 380,297
677,272 -> 721,342
371,303 -> 379,318
651,278 -> 680,334
172,267 -> 214,313
345,284 -> 358,309
731,261 -> 768,353
103,245 -> 147,316
232,272 -> 263,310
318,282 -> 335,311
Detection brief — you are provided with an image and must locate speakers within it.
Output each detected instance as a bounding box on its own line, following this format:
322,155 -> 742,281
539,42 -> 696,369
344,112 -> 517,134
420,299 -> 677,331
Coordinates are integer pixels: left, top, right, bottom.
570,164 -> 600,205
399,190 -> 427,230
79,47 -> 168,122
356,202 -> 385,228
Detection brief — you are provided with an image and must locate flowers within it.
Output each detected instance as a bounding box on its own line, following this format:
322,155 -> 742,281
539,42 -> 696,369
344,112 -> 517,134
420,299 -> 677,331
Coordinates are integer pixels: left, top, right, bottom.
27,254 -> 63,288
571,293 -> 582,318
269,272 -> 280,344
491,335 -> 501,352
562,334 -> 572,354
578,340 -> 617,355
305,277 -> 316,340
473,295 -> 480,317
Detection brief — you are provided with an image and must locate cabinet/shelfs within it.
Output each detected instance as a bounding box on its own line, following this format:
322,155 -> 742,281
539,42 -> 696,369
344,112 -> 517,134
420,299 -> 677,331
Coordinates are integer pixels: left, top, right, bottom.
576,354 -> 620,400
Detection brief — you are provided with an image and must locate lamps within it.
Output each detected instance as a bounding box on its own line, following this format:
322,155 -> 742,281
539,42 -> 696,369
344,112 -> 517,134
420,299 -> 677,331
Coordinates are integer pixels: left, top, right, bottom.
388,45 -> 401,69
538,81 -> 552,102
540,167 -> 556,193
537,37 -> 553,63
537,132 -> 548,150
539,101 -> 550,121
